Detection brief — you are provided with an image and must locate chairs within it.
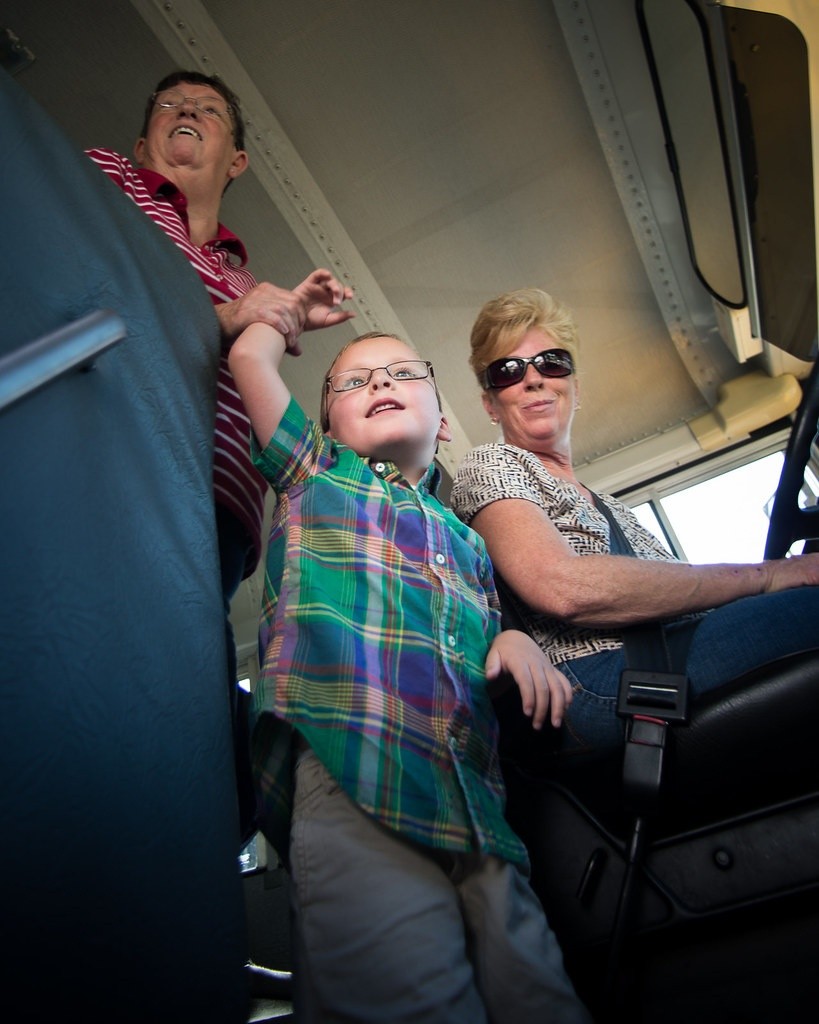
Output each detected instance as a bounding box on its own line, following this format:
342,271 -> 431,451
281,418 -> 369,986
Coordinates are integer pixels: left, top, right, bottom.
430,458 -> 819,957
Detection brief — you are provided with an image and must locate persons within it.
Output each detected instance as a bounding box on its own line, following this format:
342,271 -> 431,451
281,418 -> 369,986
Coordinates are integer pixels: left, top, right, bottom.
79,68 -> 269,739
446,289 -> 819,748
226,267 -> 586,1024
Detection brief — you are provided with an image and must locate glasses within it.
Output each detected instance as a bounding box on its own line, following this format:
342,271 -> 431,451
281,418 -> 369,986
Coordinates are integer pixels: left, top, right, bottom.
142,90 -> 238,152
324,359 -> 442,419
482,348 -> 574,389
